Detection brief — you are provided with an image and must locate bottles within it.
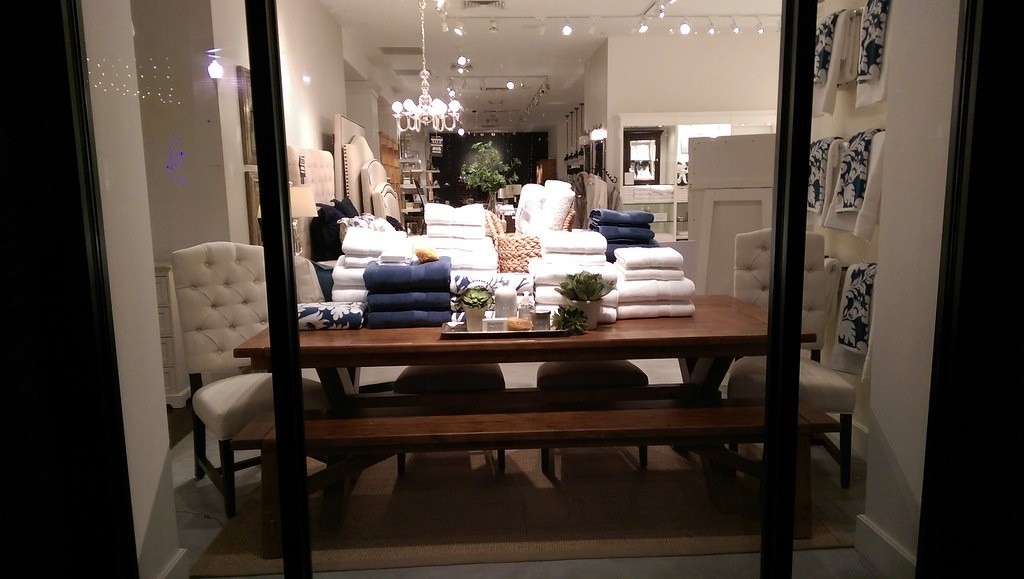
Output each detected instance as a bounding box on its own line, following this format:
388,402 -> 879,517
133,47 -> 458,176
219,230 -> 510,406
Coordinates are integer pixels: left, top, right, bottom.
519,295 -> 536,322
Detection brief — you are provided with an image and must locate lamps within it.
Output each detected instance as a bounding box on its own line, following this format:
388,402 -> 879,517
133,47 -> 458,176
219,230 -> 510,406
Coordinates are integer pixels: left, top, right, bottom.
579,123 -> 607,146
480,81 -> 550,137
391,0 -> 474,133
485,0 -> 764,35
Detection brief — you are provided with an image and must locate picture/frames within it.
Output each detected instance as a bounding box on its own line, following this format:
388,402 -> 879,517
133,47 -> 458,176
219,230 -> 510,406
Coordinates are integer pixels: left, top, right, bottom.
245,171 -> 263,244
240,65 -> 256,168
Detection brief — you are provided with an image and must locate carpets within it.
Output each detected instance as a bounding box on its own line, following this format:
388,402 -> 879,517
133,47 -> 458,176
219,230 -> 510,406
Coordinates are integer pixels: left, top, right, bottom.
189,441 -> 855,578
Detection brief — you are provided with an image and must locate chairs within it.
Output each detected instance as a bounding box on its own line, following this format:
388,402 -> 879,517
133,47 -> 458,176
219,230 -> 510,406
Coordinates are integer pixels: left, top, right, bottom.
163,240 -> 333,520
723,262 -> 886,491
730,230 -> 834,364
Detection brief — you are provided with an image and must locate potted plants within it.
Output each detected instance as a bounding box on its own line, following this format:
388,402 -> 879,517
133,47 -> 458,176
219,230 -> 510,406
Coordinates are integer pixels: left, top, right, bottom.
462,288 -> 493,333
562,271 -> 617,328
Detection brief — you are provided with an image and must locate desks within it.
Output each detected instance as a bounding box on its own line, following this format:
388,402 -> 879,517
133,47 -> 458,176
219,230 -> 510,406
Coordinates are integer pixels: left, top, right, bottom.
233,294 -> 819,491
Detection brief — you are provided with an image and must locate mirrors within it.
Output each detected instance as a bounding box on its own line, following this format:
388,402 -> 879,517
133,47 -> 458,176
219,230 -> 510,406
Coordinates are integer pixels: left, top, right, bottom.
675,123 -> 733,184
625,130 -> 663,184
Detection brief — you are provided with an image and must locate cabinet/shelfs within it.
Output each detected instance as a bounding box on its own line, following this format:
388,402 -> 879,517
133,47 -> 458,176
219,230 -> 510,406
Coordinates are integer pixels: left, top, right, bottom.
155,255 -> 191,407
402,166 -> 443,226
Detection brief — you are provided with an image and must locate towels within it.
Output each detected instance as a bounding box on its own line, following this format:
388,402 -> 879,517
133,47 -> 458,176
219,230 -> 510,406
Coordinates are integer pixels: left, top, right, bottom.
451,312 -> 535,323
450,293 -> 535,312
535,267 -> 618,286
330,180 -> 660,302
363,256 -> 452,293
367,313 -> 451,328
614,247 -> 684,280
535,287 -> 619,307
535,307 -> 617,323
616,304 -> 695,319
617,279 -> 696,303
367,291 -> 450,312
450,269 -> 536,294
823,255 -> 877,385
807,128 -> 887,242
812,0 -> 892,117
297,301 -> 367,330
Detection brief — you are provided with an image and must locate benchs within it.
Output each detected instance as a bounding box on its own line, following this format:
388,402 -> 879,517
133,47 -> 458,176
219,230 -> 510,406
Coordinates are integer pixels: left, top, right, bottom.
232,393 -> 838,567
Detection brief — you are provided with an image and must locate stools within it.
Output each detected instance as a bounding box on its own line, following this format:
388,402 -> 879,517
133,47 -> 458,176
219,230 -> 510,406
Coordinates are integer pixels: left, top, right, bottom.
536,353 -> 656,482
397,357 -> 507,476
